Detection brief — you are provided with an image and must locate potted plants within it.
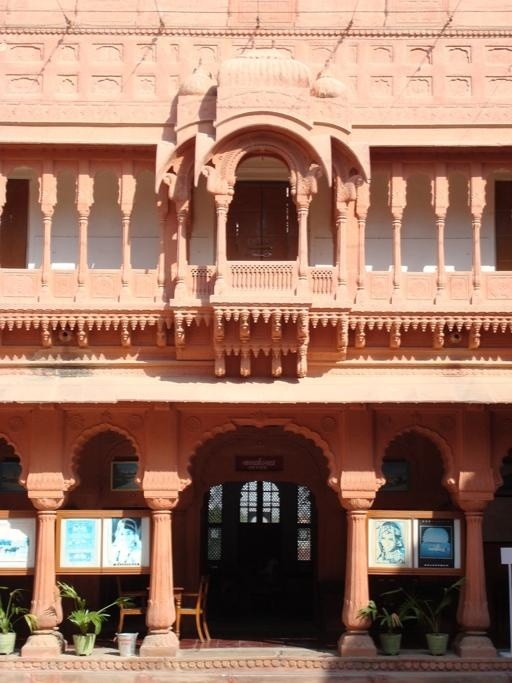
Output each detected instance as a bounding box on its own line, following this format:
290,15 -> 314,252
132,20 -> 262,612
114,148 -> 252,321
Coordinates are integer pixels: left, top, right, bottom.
358,590 -> 419,655
53,581 -> 136,656
403,577 -> 466,657
0,588 -> 38,656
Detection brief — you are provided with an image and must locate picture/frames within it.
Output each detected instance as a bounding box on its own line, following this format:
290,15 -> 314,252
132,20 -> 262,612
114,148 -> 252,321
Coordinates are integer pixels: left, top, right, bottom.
0,461 -> 27,494
494,461 -> 512,498
378,458 -> 411,493
110,460 -> 142,492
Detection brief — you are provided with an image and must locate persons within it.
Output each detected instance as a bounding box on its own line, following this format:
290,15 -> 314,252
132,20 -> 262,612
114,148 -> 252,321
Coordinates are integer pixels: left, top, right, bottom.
110,519 -> 139,565
375,525 -> 406,564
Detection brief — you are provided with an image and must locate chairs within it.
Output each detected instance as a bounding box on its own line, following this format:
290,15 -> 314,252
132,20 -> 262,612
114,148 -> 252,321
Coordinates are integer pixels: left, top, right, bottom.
110,572 -> 211,644
